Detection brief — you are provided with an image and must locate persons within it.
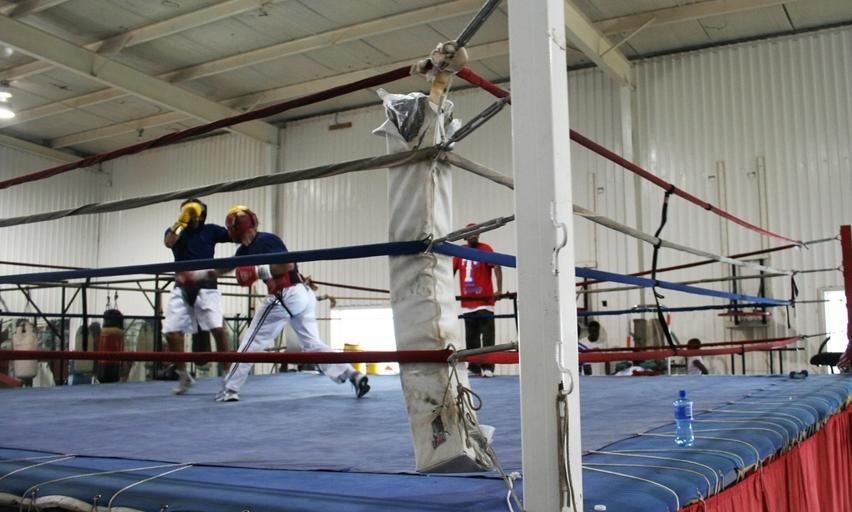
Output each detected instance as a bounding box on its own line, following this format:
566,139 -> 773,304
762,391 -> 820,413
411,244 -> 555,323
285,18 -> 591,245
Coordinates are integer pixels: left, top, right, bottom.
452,223 -> 502,378
211,206 -> 371,401
164,198 -> 242,397
578,324 -> 592,376
685,338 -> 709,376
579,321 -> 610,375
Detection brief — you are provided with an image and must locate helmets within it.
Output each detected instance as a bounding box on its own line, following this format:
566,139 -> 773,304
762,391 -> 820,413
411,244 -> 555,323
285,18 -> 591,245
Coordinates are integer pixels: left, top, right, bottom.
181,198 -> 207,225
226,206 -> 258,242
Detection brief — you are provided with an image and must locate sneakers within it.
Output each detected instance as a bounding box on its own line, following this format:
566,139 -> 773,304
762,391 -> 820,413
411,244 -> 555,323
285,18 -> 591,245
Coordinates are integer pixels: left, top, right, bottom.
350,372 -> 370,398
466,369 -> 481,377
481,368 -> 494,377
215,388 -> 238,401
174,376 -> 192,394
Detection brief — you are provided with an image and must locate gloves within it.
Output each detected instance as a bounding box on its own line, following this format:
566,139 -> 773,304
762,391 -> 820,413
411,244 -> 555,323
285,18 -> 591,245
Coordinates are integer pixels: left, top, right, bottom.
175,268 -> 217,285
236,264 -> 272,286
171,203 -> 202,236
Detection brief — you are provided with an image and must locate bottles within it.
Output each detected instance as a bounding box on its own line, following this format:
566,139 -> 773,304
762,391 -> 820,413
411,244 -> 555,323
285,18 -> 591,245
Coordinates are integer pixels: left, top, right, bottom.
672,389 -> 694,447
